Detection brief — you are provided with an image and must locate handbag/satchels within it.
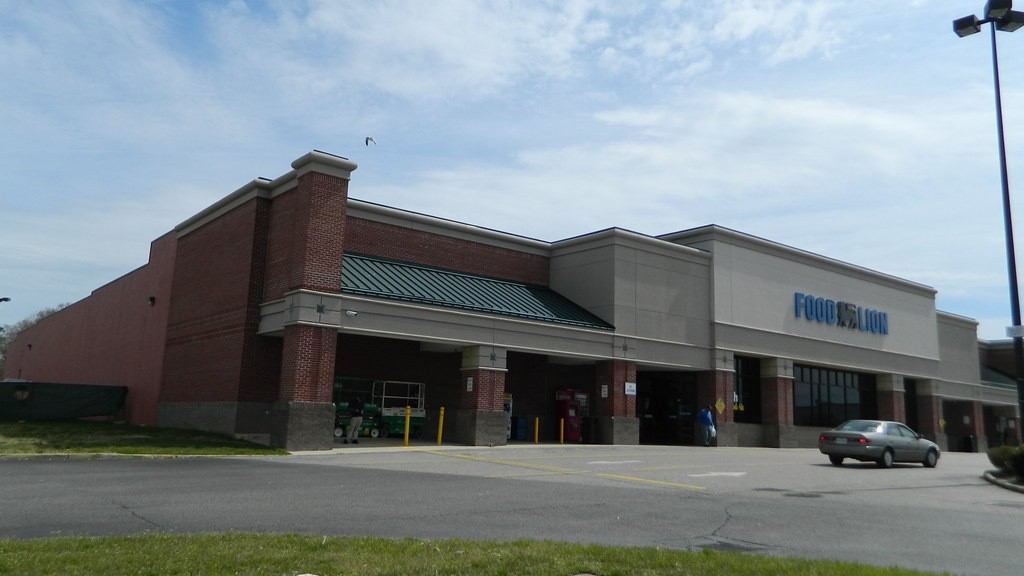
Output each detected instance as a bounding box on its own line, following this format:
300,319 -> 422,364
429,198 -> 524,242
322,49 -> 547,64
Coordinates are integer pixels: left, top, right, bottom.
709,425 -> 716,437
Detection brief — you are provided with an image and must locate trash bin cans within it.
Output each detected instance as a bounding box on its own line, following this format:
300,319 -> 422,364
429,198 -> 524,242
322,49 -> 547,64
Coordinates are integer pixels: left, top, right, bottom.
510,415 -> 530,440
963,436 -> 976,452
565,416 -> 583,442
583,417 -> 599,443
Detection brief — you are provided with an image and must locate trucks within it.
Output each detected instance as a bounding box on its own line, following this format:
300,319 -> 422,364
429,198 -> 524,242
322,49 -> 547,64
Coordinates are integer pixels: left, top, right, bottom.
331,376 -> 427,439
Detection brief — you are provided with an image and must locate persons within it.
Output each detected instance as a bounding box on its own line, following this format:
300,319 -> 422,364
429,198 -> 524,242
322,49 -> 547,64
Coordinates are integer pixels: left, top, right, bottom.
697,404 -> 714,447
343,395 -> 363,443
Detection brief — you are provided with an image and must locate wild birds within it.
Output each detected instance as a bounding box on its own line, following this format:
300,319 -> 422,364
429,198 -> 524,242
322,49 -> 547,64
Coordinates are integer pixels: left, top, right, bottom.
365,136 -> 376,146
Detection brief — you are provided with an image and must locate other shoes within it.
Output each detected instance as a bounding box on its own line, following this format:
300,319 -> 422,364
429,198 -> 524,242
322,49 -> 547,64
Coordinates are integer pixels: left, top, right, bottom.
352,440 -> 358,444
705,445 -> 711,447
343,439 -> 349,444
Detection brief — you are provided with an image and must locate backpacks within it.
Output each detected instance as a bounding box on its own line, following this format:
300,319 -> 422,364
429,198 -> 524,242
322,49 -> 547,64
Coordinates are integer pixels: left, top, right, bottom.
697,409 -> 711,425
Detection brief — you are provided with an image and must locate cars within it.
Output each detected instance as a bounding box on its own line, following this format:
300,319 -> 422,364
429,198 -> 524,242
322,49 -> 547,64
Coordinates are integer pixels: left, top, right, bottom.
818,419 -> 941,469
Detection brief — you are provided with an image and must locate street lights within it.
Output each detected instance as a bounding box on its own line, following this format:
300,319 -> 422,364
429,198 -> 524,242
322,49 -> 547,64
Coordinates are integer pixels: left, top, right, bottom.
952,1 -> 1024,482
